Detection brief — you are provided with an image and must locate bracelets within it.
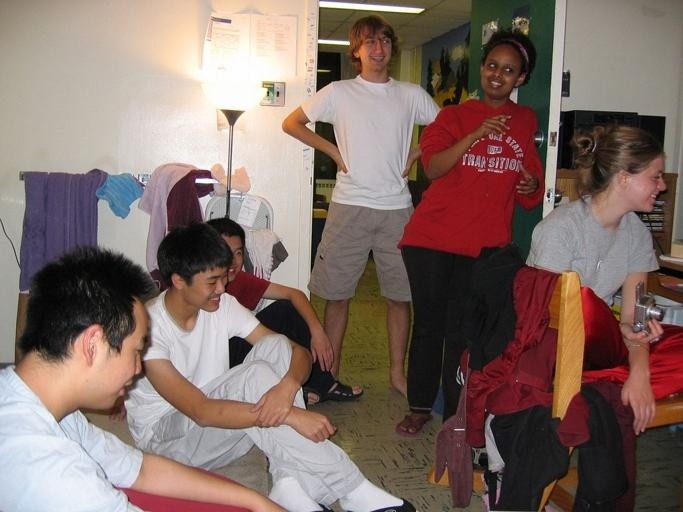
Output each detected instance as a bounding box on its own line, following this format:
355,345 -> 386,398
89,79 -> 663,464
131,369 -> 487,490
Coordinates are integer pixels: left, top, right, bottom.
627,342 -> 650,354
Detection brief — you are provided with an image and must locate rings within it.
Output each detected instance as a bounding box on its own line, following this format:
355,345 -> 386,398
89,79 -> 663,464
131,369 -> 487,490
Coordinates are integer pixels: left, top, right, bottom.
649,336 -> 660,344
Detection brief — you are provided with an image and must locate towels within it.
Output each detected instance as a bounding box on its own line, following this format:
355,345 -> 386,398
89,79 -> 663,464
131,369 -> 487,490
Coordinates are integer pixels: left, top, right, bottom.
18,163 -> 213,292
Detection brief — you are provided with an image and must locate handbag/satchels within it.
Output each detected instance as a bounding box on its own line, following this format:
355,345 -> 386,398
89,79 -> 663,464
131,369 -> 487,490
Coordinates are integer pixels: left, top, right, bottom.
433,415 -> 474,508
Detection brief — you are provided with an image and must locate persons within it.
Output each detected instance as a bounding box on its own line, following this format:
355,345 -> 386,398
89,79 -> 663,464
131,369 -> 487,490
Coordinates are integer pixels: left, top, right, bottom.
0,241 -> 291,512
393,30 -> 546,438
202,217 -> 363,407
279,14 -> 441,401
470,122 -> 669,511
122,217 -> 420,512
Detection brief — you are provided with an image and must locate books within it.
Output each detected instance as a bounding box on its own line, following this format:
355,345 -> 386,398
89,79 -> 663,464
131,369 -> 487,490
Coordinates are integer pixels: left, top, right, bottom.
658,253 -> 683,263
640,199 -> 666,233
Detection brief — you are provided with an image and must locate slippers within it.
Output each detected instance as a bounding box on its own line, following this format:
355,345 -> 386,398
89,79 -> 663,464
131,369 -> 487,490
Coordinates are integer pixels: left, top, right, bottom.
394,413 -> 433,437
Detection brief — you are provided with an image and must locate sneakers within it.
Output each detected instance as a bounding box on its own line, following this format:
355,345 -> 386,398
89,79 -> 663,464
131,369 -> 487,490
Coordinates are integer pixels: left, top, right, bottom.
312,502 -> 335,511
346,497 -> 417,512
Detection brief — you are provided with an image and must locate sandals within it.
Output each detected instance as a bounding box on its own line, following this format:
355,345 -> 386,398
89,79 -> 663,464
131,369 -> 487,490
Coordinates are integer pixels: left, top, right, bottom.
320,379 -> 363,403
302,386 -> 322,407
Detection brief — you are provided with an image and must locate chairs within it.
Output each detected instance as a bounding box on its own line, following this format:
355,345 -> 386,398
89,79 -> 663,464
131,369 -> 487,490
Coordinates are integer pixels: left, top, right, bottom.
428,270 -> 682,512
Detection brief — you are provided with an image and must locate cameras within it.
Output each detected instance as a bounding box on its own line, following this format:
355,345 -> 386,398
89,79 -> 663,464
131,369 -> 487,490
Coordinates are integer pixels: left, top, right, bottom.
633,280 -> 664,333
